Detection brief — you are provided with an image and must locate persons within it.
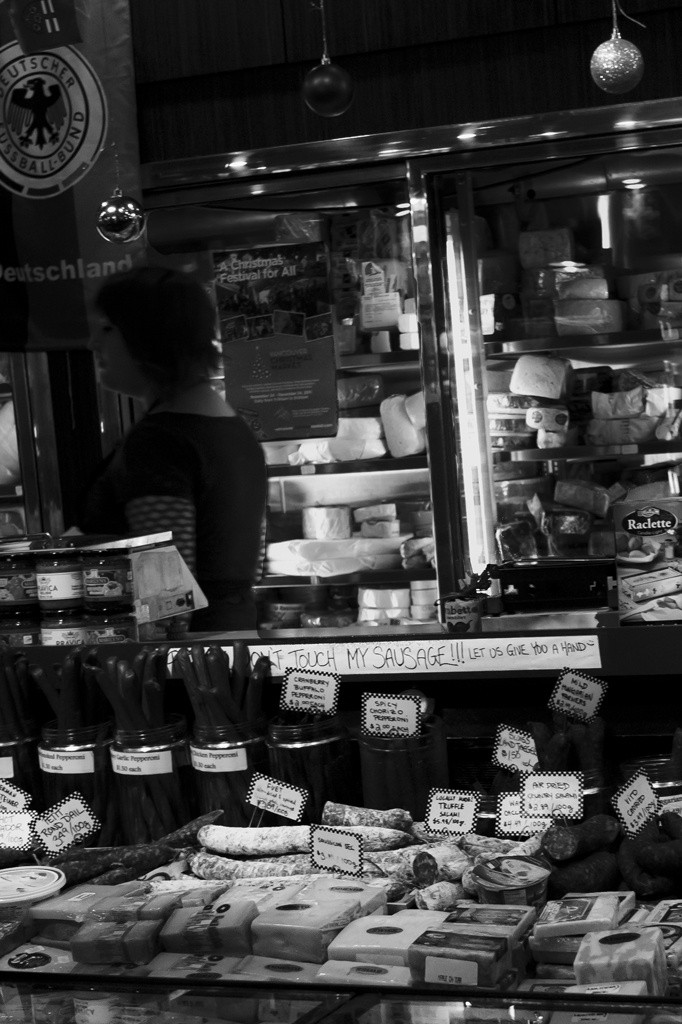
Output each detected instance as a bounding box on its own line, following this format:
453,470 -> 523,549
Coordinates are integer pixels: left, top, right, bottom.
73,266 -> 268,631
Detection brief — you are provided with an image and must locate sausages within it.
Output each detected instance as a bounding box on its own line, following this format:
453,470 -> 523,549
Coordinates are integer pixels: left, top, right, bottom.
58,802 -> 543,912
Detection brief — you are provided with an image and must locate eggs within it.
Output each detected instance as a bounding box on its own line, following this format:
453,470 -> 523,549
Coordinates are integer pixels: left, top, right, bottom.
616,533 -> 656,559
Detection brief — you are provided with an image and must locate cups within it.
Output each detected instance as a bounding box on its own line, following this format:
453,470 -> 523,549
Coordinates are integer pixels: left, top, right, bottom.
30,994 -> 118,1024
4,720 -> 439,849
474,757 -> 682,838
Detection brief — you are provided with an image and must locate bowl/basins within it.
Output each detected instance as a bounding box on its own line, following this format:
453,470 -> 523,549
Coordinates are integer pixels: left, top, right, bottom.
260,390 -> 437,629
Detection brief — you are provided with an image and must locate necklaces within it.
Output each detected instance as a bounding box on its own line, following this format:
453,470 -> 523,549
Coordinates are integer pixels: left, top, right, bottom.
133,375 -> 210,422
